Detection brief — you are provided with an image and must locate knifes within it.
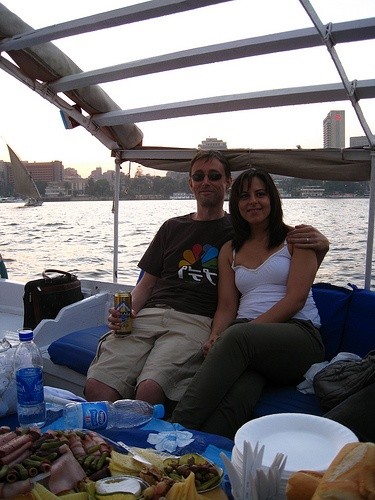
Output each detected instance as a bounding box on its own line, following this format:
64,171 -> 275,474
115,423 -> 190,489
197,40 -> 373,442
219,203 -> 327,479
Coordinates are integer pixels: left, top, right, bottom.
212,442 -> 287,500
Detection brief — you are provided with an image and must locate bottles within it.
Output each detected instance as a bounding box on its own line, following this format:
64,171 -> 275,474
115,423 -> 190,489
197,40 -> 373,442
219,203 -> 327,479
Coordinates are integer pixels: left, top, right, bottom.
16,327 -> 48,430
61,399 -> 167,430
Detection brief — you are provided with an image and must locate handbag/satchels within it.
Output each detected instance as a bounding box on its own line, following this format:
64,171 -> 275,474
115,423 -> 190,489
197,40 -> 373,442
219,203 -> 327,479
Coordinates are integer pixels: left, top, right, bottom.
22,269 -> 82,329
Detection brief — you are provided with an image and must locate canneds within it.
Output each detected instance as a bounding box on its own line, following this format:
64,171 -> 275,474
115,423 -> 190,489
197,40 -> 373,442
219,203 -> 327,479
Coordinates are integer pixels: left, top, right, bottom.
113,291 -> 132,336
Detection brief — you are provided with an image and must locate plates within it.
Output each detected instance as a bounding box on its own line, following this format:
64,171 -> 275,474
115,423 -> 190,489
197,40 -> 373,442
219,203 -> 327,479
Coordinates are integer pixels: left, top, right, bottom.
228,413 -> 360,498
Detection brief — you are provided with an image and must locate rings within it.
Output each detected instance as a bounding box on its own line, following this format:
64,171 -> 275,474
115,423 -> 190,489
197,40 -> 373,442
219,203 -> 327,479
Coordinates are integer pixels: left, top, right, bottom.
306,236 -> 311,244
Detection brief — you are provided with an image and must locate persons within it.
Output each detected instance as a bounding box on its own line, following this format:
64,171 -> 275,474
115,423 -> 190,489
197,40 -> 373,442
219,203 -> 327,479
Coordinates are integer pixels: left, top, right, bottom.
80,146 -> 329,418
169,166 -> 325,432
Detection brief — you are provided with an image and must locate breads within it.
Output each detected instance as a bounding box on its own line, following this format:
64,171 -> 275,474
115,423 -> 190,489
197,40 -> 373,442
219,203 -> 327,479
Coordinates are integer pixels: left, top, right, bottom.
287,442 -> 375,499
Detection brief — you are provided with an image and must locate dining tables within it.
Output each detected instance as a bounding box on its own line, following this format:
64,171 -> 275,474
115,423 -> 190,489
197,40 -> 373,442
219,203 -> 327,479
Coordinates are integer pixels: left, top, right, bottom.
0,404 -> 234,500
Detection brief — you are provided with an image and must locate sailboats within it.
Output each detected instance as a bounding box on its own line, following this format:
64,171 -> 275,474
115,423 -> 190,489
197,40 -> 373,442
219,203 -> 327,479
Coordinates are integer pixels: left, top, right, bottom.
5,144 -> 43,206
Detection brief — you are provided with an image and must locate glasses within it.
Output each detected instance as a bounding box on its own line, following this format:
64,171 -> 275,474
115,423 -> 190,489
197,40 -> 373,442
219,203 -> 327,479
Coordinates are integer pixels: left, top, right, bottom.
191,173 -> 220,181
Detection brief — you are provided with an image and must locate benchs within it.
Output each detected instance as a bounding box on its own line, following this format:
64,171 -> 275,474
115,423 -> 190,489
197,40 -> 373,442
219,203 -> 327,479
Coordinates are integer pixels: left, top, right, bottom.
47,288 -> 375,420
